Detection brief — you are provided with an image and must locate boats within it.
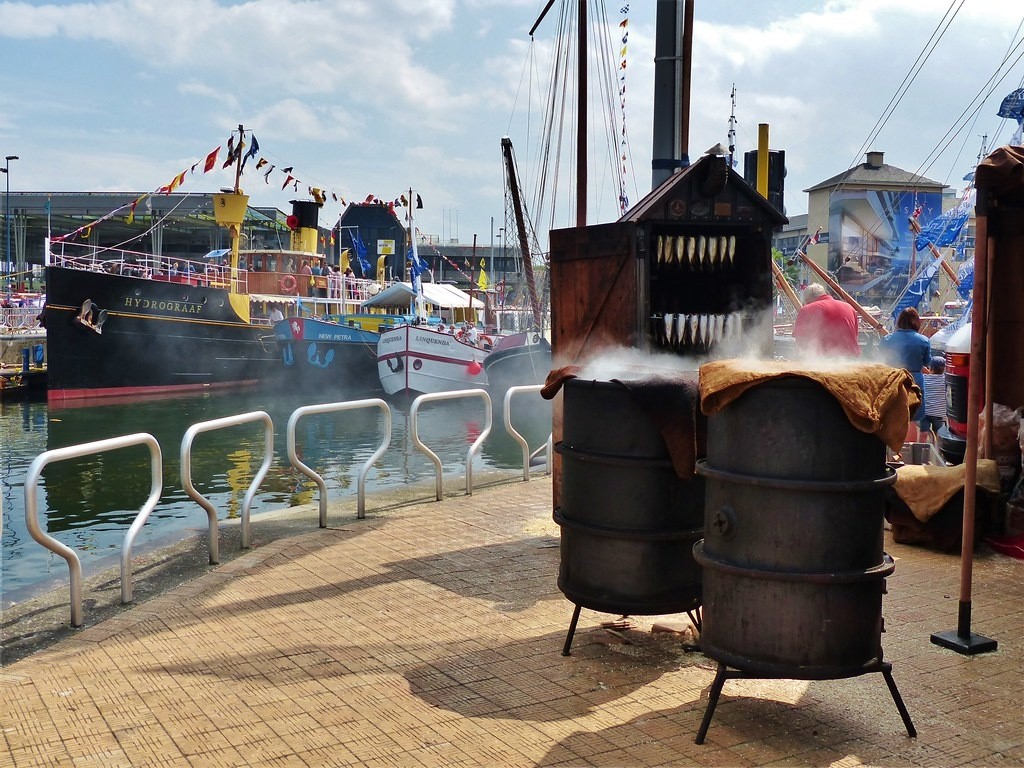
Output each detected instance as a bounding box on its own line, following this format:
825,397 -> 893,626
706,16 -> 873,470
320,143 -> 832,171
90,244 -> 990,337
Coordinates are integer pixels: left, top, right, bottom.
267,288 -> 446,392
375,289 -> 517,412
40,123 -> 491,413
480,1 -> 665,464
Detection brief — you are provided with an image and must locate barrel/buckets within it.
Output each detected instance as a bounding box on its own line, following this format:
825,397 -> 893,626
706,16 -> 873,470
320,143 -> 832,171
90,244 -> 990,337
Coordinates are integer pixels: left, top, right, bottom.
554,377 -> 710,615
943,352 -> 983,439
700,371 -> 885,676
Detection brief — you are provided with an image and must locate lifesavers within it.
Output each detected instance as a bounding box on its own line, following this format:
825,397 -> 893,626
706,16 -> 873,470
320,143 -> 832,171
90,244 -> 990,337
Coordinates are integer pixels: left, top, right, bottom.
280,275 -> 296,293
479,336 -> 493,349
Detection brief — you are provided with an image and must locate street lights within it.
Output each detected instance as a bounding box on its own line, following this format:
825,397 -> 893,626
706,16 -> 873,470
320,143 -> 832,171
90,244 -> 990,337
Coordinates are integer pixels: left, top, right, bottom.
0,153 -> 19,302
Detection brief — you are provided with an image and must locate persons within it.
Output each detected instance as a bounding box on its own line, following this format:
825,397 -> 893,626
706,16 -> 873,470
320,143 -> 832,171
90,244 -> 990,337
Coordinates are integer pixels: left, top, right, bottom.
879,306 -> 932,422
792,283 -> 860,362
207,259 -> 215,272
102,263 -> 164,279
918,356 -> 947,443
312,262 -> 320,275
3,295 -> 36,326
170,262 -> 178,276
437,323 -> 477,348
301,261 -> 313,275
218,259 -> 227,272
249,264 -> 255,272
183,261 -> 195,277
285,259 -> 293,273
240,257 -> 246,269
269,304 -> 284,325
321,262 -> 359,299
